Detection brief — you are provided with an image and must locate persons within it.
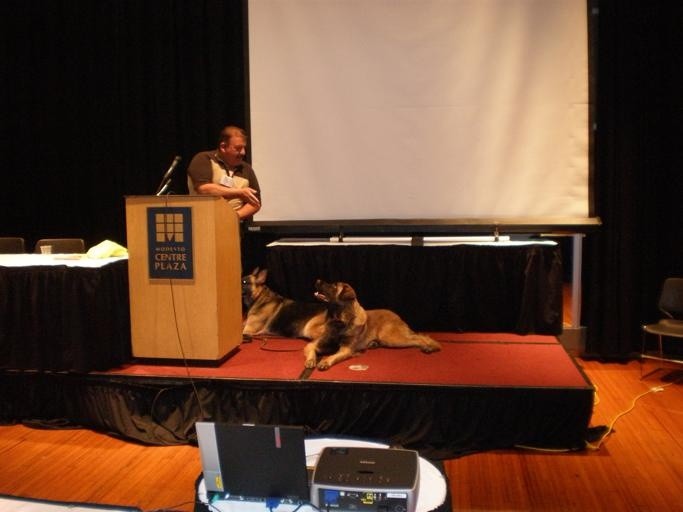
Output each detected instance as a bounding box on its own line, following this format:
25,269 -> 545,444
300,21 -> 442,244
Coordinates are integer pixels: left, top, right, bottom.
187,125 -> 261,233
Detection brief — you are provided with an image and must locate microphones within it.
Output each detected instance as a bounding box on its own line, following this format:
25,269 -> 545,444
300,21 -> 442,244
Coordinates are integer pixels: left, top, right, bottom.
163,155 -> 181,182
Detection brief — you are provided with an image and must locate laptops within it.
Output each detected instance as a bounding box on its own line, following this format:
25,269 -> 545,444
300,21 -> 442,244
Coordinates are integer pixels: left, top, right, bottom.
195,422 -> 314,503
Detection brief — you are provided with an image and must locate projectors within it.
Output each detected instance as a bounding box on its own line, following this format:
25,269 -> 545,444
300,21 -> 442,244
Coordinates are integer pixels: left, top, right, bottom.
311,447 -> 419,512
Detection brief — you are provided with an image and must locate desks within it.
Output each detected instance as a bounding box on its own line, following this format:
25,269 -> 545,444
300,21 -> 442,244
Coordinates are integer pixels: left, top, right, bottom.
0,251 -> 133,372
190,434 -> 452,512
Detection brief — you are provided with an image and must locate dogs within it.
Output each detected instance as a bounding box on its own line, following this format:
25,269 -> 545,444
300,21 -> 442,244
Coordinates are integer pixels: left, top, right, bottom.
303,279 -> 442,372
241,265 -> 330,339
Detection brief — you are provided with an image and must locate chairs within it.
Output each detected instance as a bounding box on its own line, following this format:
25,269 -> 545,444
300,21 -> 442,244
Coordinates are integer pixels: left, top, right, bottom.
32,238 -> 87,253
640,277 -> 683,380
0,237 -> 25,253
